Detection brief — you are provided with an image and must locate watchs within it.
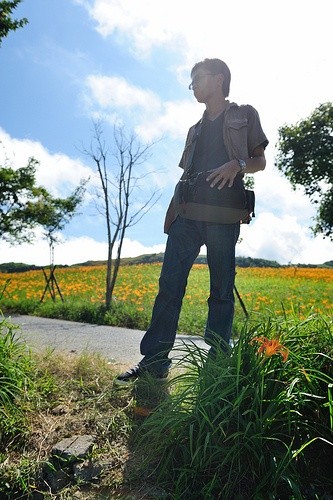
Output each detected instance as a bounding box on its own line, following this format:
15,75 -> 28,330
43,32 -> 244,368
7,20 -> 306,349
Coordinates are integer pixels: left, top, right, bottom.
237,158 -> 247,174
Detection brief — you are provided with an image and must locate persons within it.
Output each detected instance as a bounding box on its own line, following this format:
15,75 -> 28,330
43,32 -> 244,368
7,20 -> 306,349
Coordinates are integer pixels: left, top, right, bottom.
114,56 -> 267,385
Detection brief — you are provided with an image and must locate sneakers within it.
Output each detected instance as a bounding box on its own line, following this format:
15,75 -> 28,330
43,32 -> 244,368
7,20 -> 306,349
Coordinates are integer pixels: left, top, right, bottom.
117,359 -> 172,381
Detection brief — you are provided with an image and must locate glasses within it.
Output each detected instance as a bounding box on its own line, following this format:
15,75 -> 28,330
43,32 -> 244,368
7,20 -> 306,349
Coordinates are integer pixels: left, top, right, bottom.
189,73 -> 215,90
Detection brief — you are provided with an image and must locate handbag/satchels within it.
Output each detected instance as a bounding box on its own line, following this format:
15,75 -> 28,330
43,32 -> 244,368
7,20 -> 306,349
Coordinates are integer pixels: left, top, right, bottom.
180,170 -> 255,225
